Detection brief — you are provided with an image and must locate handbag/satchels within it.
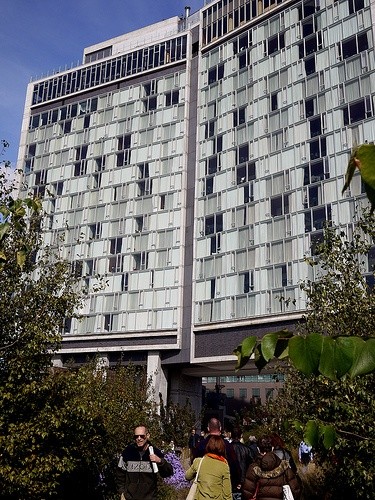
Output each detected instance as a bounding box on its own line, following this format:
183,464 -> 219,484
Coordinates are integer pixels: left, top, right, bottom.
282,485 -> 294,500
186,456 -> 205,500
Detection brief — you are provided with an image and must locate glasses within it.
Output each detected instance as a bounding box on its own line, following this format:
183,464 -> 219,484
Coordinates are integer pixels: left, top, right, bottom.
134,434 -> 147,438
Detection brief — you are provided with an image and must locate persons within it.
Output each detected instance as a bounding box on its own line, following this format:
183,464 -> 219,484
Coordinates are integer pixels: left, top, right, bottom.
116,425 -> 173,500
298,440 -> 313,466
185,418 -> 302,500
